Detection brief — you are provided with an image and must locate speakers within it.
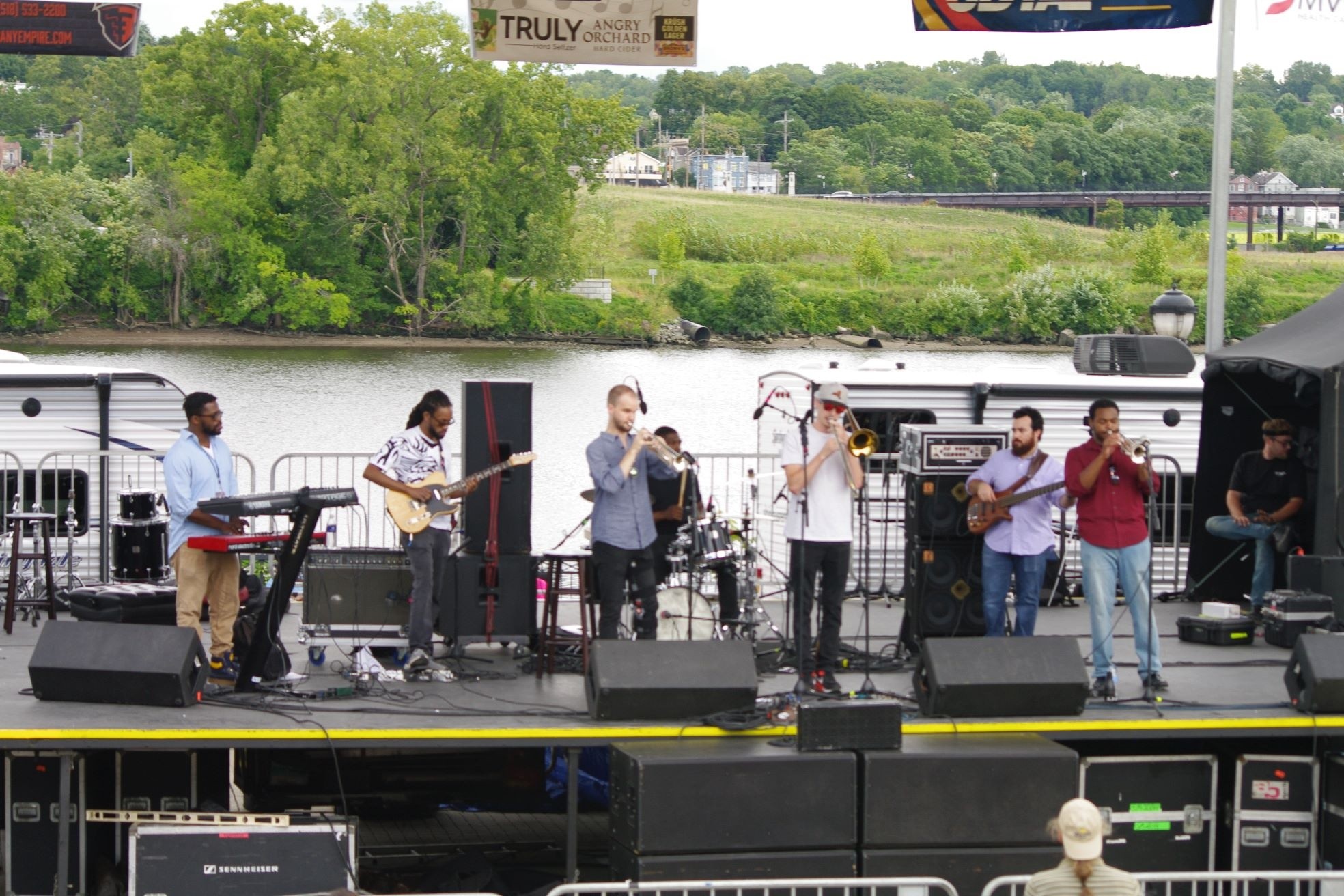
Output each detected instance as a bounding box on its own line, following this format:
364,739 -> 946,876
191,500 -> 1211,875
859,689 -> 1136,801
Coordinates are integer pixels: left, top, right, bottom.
1283,630 -> 1344,714
306,547 -> 416,635
913,635 -> 1089,720
459,380 -> 531,553
583,637 -> 759,720
1286,554 -> 1344,627
26,619 -> 210,709
126,818 -> 357,896
899,472 -> 993,651
444,551 -> 532,643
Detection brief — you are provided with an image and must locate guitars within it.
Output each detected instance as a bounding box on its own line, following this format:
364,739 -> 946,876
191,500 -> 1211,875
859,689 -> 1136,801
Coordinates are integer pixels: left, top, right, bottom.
967,475 -> 1066,533
386,451 -> 539,534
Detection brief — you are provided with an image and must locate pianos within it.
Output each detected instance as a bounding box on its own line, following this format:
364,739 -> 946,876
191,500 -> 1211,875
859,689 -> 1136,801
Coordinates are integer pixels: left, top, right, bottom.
186,485 -> 360,690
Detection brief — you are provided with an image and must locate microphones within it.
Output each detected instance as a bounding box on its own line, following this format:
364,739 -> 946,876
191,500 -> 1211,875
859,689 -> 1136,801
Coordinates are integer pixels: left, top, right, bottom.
754,388 -> 776,420
635,378 -> 647,415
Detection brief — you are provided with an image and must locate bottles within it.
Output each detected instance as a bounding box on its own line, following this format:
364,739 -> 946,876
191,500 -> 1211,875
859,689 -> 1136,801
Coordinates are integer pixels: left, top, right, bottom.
326,514 -> 336,548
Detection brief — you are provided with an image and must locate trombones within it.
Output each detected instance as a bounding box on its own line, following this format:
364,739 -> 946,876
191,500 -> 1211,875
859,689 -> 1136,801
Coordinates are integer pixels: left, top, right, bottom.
830,407 -> 880,496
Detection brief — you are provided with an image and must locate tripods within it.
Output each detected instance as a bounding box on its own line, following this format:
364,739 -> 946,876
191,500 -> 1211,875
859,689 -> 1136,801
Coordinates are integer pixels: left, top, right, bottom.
756,482 -> 800,661
752,414 -> 846,701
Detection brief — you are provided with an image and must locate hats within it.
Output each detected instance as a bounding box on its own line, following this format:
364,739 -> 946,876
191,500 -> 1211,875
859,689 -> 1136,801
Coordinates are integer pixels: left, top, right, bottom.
814,382 -> 851,407
1057,797 -> 1104,861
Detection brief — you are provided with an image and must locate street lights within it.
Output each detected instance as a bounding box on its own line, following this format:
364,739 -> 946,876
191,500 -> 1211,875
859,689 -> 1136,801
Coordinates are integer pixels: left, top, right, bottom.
817,173 -> 825,196
1084,197 -> 1098,228
1310,199 -> 1318,240
1082,170 -> 1087,191
992,173 -> 996,195
908,173 -> 913,195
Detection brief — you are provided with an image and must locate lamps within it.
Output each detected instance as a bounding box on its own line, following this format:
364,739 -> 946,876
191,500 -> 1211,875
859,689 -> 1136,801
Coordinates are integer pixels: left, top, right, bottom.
1149,280 -> 1199,343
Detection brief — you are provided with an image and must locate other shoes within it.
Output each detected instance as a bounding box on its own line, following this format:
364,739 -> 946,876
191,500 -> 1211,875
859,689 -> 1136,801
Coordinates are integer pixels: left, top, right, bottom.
819,649 -> 862,668
1143,674 -> 1169,687
1247,605 -> 1264,621
208,651 -> 238,685
1093,675 -> 1115,695
1273,525 -> 1294,551
403,654 -> 453,679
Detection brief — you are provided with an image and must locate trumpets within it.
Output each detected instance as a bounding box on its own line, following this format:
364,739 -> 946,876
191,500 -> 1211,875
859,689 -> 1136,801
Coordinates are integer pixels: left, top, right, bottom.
1107,430 -> 1147,464
628,421 -> 687,472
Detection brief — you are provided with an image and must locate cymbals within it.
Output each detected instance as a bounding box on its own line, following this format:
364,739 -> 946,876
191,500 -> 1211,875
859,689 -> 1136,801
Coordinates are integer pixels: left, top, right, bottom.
725,470 -> 786,485
581,489 -> 595,503
715,512 -> 785,521
579,545 -> 592,550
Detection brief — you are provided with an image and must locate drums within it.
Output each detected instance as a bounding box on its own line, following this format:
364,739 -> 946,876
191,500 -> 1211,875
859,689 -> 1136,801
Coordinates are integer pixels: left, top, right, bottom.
108,488 -> 171,583
617,590 -> 634,639
634,584 -> 717,641
664,532 -> 691,561
677,516 -> 735,568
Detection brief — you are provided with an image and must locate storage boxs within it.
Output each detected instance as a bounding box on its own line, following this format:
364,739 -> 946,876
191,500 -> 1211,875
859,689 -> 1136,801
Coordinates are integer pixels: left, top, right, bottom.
1258,606 -> 1337,650
1201,602 -> 1241,620
3,749 -> 85,896
296,562 -> 411,648
1079,753 -> 1219,896
127,821 -> 356,896
1175,615 -> 1255,648
1229,752 -> 1321,896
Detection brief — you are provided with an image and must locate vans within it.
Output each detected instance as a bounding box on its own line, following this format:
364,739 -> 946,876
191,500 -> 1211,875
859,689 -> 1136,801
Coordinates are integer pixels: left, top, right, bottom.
831,191 -> 852,195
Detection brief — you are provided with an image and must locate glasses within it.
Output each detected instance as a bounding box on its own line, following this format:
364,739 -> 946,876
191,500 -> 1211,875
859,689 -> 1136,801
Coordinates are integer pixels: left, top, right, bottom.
201,411 -> 223,419
1272,436 -> 1294,447
822,402 -> 847,415
432,416 -> 455,427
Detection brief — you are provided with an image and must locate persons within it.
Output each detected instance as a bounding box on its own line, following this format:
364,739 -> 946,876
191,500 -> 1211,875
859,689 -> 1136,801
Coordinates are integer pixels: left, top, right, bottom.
650,425 -> 743,632
160,393 -> 242,680
585,384 -> 679,638
782,383 -> 863,691
1065,399 -> 1170,696
1204,417 -> 1307,620
965,406 -> 1075,636
362,389 -> 479,674
1023,797 -> 1142,895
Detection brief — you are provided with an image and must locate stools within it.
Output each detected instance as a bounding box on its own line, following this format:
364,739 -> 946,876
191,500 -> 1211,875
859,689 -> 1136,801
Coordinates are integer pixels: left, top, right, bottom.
536,551 -> 596,680
68,582 -> 177,626
3,512 -> 57,636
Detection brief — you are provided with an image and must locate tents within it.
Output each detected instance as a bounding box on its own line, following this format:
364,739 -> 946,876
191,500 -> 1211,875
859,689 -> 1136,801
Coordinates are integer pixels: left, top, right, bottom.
1181,288 -> 1344,601
